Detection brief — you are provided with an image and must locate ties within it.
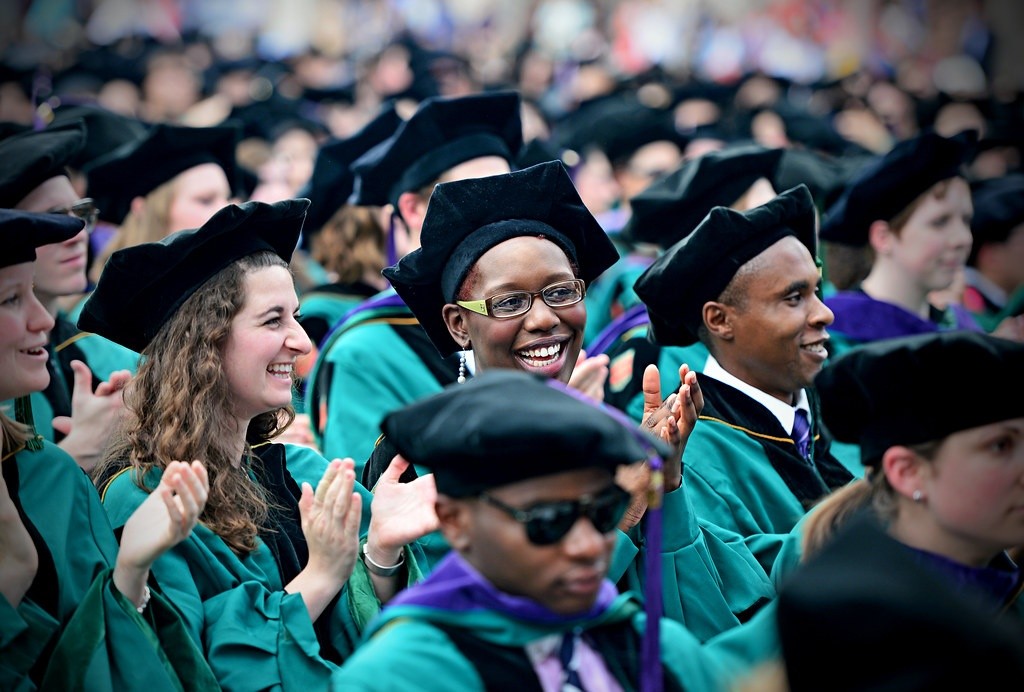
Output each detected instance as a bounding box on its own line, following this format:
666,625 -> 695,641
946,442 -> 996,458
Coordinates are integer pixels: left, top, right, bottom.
792,411 -> 819,467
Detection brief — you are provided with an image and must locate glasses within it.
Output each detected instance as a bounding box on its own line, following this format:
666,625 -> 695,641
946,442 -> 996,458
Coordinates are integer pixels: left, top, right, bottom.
46,199 -> 97,227
482,484 -> 633,546
456,278 -> 584,319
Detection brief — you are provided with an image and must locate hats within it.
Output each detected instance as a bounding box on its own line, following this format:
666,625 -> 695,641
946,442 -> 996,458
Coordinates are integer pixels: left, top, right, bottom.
78,197 -> 311,354
84,117 -> 239,223
380,372 -> 673,499
347,92 -> 524,209
617,141 -> 785,249
381,160 -> 621,357
0,132 -> 82,207
635,183 -> 817,348
0,209 -> 86,268
970,175 -> 1024,245
294,101 -> 408,251
817,128 -> 981,245
813,335 -> 1024,445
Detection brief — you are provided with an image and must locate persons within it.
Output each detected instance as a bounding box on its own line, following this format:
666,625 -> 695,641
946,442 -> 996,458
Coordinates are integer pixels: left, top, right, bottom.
0,29 -> 522,484
0,159 -> 744,692
501,31 -> 1024,421
633,182 -> 1024,692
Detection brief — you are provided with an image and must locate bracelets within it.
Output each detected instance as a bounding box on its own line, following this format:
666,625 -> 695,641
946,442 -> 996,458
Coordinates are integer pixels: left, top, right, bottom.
363,543 -> 405,577
137,584 -> 150,613
